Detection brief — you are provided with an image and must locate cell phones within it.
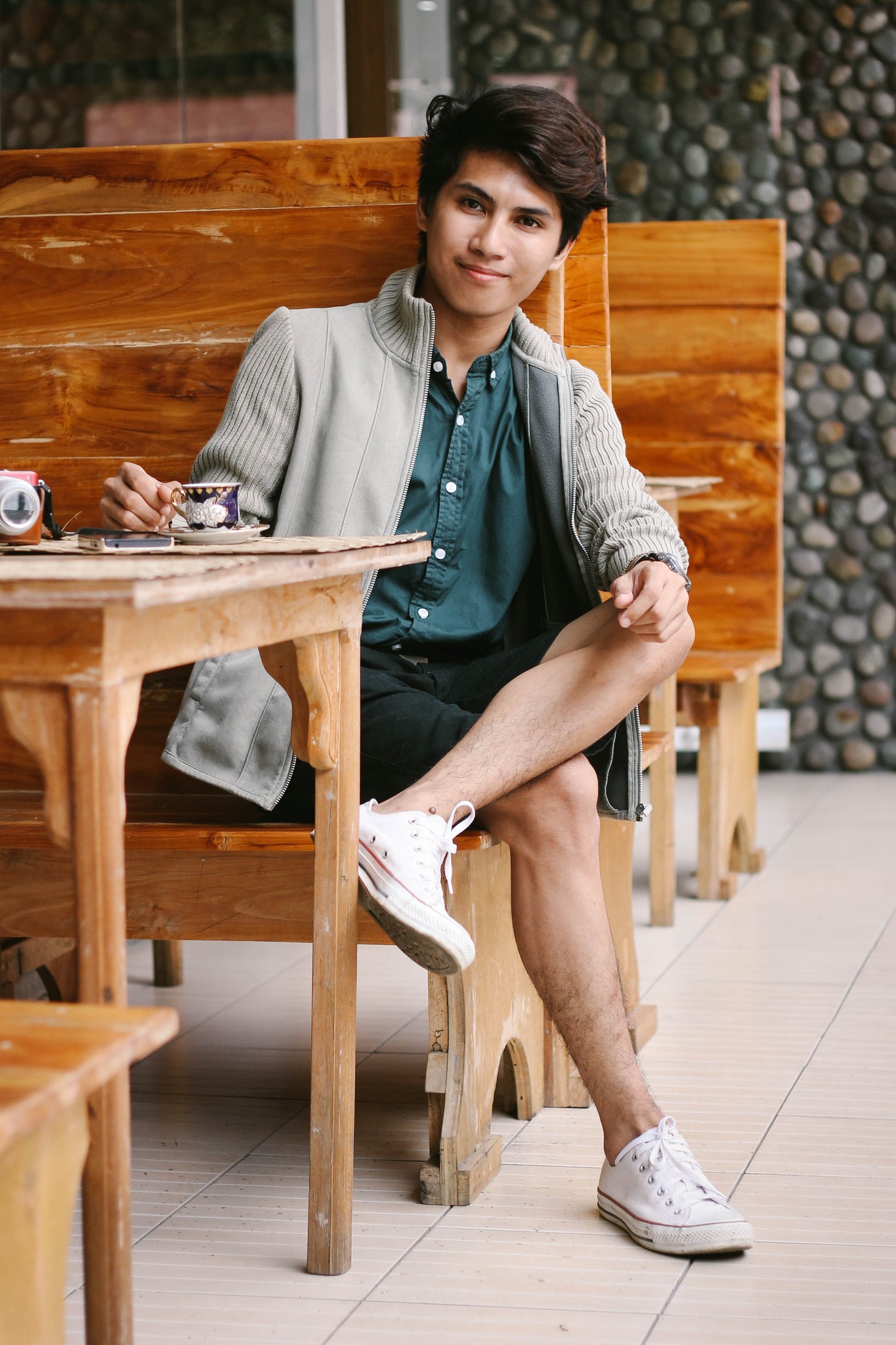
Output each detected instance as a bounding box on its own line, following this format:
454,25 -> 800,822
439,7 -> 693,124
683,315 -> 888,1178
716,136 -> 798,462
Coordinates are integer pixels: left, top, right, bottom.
78,535 -> 176,551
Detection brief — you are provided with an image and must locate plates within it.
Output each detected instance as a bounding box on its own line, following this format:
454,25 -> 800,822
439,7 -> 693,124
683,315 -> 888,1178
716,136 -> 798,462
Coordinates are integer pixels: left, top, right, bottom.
157,523 -> 272,544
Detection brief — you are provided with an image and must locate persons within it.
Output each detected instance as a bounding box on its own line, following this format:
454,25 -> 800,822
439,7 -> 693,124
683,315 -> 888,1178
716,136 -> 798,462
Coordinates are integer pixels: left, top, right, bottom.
100,84 -> 754,1255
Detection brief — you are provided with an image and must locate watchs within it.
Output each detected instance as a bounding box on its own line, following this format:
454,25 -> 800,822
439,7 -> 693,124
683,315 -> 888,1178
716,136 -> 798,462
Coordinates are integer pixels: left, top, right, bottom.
624,551 -> 692,595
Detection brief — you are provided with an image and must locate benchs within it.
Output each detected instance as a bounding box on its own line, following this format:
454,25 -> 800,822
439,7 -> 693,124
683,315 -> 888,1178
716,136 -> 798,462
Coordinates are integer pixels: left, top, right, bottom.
0,137 -> 788,1204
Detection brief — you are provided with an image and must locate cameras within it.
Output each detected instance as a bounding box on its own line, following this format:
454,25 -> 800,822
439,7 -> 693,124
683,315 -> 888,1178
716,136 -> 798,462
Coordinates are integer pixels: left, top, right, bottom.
0,469 -> 45,543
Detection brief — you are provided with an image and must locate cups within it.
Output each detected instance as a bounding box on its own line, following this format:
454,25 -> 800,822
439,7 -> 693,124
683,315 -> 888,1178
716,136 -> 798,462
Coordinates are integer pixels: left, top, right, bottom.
171,483 -> 241,532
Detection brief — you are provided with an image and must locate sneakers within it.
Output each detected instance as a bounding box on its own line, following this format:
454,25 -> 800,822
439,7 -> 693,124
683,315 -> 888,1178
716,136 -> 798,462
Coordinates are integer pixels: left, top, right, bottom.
597,1114 -> 754,1254
309,798 -> 477,977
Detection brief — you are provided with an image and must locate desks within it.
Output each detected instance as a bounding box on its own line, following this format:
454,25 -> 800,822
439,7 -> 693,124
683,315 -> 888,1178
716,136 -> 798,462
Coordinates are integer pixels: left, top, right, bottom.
0,528 -> 433,1345
